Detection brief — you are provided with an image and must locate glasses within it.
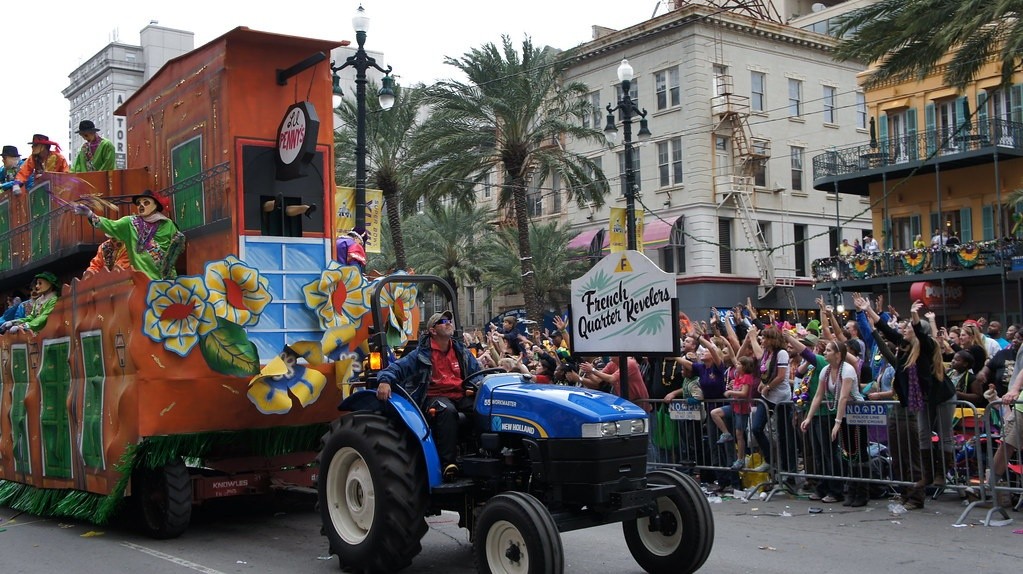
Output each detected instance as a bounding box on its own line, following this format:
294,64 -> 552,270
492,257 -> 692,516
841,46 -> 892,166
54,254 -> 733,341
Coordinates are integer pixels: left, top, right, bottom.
436,319 -> 452,324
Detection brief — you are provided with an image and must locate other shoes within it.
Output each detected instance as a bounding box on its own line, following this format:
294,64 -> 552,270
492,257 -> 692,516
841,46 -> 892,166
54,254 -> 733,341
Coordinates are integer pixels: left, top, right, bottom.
443,463 -> 459,478
713,484 -> 726,491
965,487 -> 990,501
895,498 -> 923,510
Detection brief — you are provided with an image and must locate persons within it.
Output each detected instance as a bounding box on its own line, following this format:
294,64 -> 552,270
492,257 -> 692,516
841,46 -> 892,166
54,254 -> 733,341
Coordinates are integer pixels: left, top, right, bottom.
914,228 -> 960,274
86,233 -> 131,273
0,271 -> 62,336
75,189 -> 185,280
70,120 -> 115,173
0,143 -> 34,191
463,292 -> 1023,509
840,234 -> 882,279
377,310 -> 483,483
13,134 -> 69,196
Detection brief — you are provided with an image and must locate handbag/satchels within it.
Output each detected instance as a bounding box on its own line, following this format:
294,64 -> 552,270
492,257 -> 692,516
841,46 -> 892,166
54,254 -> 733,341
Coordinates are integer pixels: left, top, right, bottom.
652,401 -> 680,449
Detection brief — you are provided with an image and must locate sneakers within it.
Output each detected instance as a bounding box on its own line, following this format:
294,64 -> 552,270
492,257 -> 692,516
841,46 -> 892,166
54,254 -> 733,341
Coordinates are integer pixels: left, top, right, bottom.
755,462 -> 776,472
731,459 -> 744,469
808,492 -> 821,499
716,433 -> 734,444
822,495 -> 837,503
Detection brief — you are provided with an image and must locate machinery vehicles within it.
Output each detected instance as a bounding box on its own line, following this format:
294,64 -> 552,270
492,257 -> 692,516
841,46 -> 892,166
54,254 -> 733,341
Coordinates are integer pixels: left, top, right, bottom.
0,24 -> 715,574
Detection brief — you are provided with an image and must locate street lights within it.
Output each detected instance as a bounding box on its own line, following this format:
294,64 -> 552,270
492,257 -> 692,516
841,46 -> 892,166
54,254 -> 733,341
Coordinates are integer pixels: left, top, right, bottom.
330,3 -> 397,251
603,54 -> 652,250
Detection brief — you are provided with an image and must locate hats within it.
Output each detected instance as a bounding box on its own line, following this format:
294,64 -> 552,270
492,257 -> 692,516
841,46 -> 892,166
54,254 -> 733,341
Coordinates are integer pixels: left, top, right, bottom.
28,134 -> 56,145
549,330 -> 563,337
0,146 -> 22,157
427,310 -> 452,334
132,189 -> 163,212
799,334 -> 818,346
75,120 -> 100,133
34,271 -> 61,290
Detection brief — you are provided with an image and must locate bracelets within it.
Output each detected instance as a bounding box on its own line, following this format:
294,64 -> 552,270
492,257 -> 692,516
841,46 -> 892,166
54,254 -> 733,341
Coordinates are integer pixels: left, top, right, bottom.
696,335 -> 700,340
766,385 -> 770,390
834,419 -> 842,423
592,368 -> 594,373
488,346 -> 494,350
579,377 -> 582,382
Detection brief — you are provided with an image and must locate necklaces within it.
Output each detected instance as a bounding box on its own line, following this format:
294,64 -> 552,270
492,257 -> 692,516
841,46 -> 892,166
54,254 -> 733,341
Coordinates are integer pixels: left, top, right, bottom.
662,359 -> 677,385
136,219 -> 159,254
160,234 -> 185,279
825,365 -> 841,411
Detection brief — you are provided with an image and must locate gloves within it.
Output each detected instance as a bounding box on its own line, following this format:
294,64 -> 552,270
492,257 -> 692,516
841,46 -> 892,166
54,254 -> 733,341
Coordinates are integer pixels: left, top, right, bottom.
71,204 -> 94,219
0,321 -> 12,330
9,325 -> 24,334
13,184 -> 22,197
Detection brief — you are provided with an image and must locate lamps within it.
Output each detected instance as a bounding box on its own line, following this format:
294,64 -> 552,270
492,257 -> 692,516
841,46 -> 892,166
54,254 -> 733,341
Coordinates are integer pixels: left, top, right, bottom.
663,200 -> 670,209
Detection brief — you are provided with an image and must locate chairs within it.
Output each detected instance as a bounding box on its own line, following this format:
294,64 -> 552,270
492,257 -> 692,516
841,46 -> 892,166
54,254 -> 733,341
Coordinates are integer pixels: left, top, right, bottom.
867,408 -> 1023,511
938,136 -> 955,154
834,147 -> 898,173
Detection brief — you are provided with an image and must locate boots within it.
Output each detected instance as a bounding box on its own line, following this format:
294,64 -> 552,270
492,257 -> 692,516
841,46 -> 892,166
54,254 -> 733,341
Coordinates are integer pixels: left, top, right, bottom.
842,465 -> 858,506
914,449 -> 936,489
933,449 -> 952,487
851,467 -> 868,507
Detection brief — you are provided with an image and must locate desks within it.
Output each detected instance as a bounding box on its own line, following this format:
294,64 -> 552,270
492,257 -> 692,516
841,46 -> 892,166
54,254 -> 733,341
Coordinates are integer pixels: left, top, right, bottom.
953,135 -> 987,153
861,152 -> 890,167
